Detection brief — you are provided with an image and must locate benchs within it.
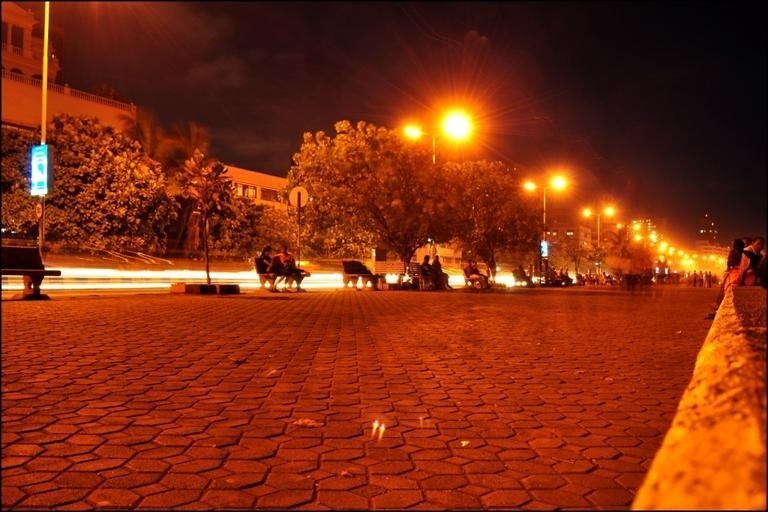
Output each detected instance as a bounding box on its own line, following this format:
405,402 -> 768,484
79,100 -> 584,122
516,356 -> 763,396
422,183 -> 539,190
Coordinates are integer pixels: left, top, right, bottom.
408,262 -> 430,291
342,260 -> 384,291
253,258 -> 311,292
0,245 -> 62,300
462,268 -> 476,290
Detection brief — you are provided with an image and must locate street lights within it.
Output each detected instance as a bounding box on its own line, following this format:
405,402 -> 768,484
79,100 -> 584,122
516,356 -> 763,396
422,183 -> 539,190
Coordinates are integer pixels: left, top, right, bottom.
579,205 -> 725,278
523,175 -> 568,240
399,106 -> 478,163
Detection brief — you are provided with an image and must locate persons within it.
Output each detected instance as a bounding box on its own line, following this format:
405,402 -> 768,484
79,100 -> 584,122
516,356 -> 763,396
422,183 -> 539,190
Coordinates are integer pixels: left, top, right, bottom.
432,256 -> 451,290
421,255 -> 443,290
465,260 -> 613,289
704,237 -> 768,319
260,245 -> 285,292
273,244 -> 304,292
686,269 -> 712,289
